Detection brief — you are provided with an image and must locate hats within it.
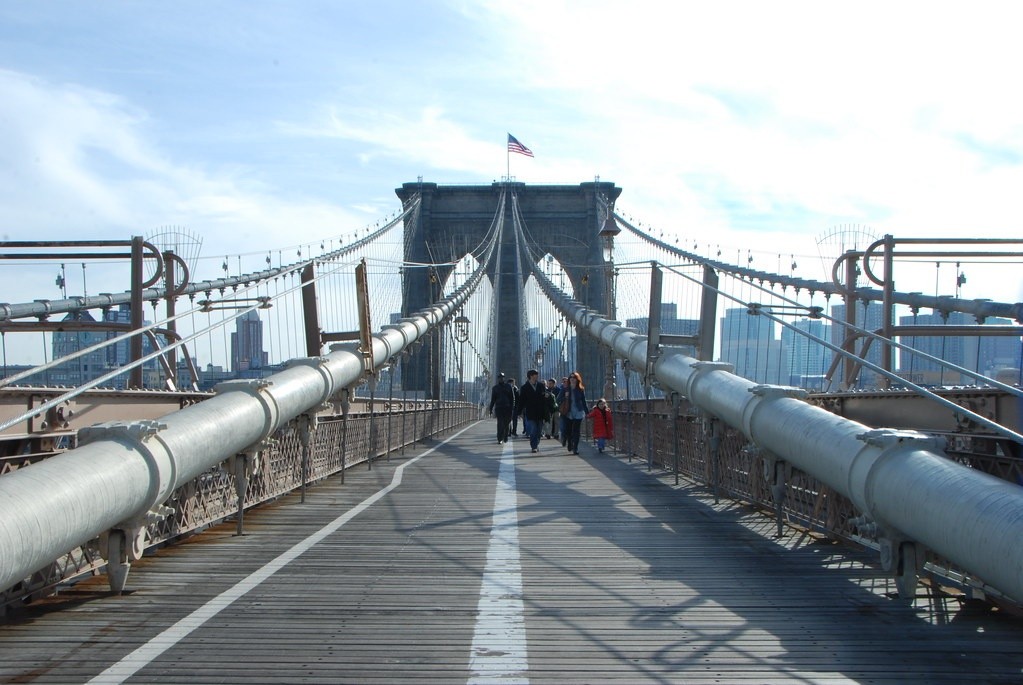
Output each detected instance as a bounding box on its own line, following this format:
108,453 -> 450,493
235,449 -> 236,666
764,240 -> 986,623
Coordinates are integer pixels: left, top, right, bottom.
498,372 -> 505,377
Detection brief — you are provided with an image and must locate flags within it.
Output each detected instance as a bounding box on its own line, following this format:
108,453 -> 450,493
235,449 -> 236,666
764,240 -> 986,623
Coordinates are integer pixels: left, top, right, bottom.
508,133 -> 534,158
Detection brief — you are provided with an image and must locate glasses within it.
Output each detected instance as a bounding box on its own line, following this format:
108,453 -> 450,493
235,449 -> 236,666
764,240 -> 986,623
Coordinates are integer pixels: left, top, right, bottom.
570,376 -> 576,379
562,381 -> 568,383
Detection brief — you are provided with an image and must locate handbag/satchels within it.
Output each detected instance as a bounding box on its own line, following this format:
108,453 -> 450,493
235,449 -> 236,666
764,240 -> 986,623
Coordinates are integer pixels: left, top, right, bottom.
560,387 -> 570,415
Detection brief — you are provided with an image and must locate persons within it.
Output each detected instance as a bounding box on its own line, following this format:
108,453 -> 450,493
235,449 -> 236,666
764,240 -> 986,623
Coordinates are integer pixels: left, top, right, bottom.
585,399 -> 613,453
518,370 -> 588,455
490,373 -> 519,444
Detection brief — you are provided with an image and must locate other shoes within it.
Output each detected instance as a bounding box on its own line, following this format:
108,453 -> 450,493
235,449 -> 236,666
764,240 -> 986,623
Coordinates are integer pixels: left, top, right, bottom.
504,436 -> 508,443
537,447 -> 540,451
599,448 -> 603,453
508,430 -> 560,440
562,440 -> 566,446
532,448 -> 537,452
573,448 -> 579,454
567,447 -> 573,451
498,440 -> 503,444
